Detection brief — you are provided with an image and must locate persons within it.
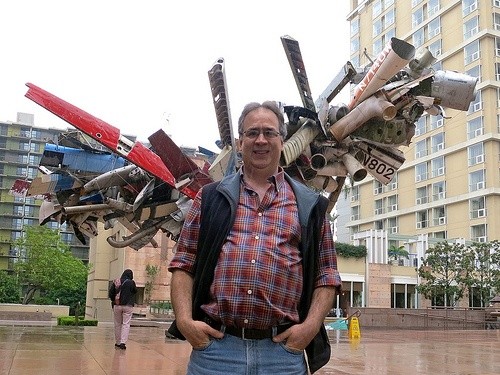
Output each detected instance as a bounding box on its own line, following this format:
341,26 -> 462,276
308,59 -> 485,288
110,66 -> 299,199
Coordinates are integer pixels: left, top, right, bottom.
168,101 -> 343,375
108,269 -> 137,350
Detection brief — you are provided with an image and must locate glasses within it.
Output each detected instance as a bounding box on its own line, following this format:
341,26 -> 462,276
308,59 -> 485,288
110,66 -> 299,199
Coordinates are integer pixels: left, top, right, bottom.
242,126 -> 281,141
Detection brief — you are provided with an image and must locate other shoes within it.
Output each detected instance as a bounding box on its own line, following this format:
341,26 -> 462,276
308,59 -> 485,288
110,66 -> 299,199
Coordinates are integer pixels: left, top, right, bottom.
115,343 -> 122,347
121,343 -> 126,349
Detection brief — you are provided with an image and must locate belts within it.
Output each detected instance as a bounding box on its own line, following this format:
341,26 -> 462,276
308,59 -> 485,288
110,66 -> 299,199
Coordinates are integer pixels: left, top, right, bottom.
207,320 -> 294,340
